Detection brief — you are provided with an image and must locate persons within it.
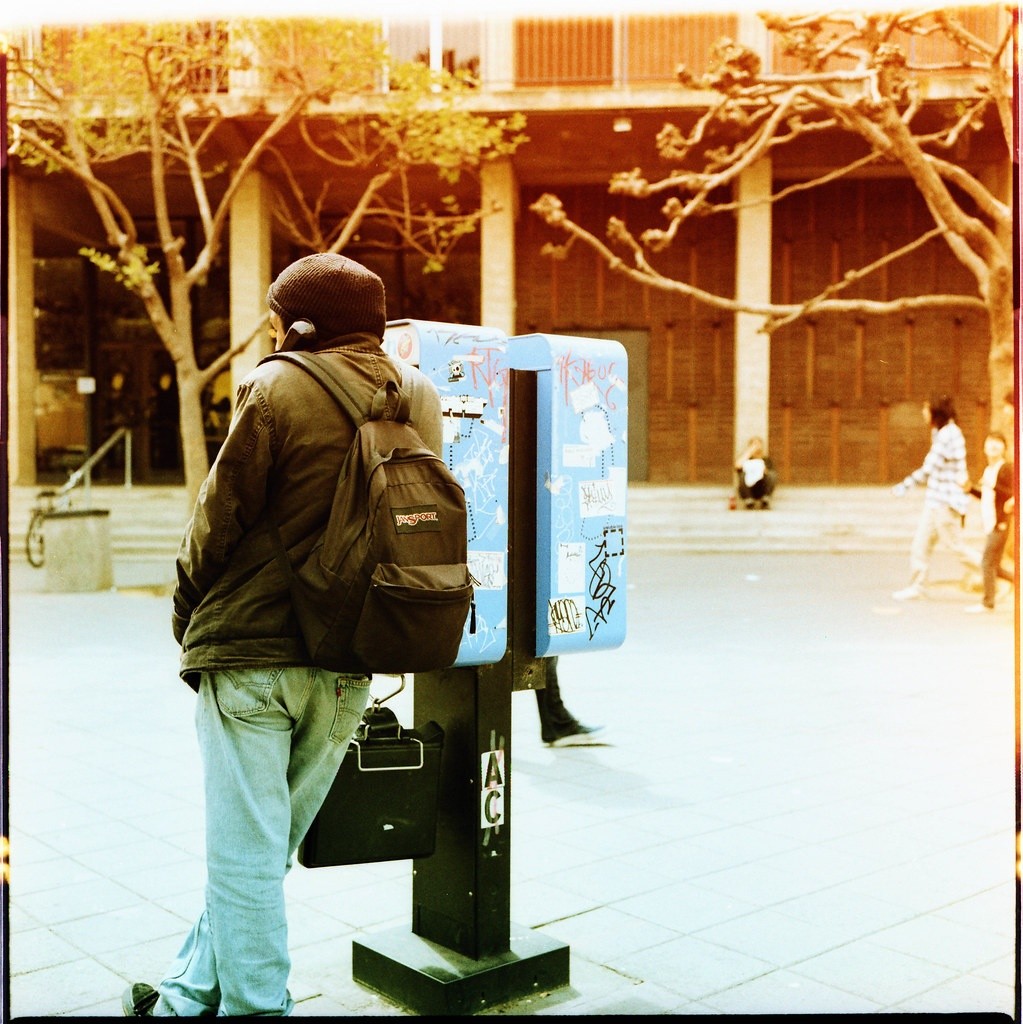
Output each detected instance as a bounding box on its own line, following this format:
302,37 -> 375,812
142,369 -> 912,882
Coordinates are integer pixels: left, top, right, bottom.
537,657 -> 609,749
963,432 -> 1016,612
891,394 -> 970,601
735,436 -> 776,509
120,253 -> 442,1017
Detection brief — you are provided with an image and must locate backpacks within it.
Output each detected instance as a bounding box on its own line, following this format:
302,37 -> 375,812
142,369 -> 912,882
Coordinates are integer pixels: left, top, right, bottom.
254,345 -> 478,679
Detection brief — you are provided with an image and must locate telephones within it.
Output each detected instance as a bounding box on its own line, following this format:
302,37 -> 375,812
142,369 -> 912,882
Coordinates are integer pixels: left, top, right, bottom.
280,320 -> 317,351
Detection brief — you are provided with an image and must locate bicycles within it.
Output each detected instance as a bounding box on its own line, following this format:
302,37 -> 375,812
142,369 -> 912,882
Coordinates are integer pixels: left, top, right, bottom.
26,468 -> 77,568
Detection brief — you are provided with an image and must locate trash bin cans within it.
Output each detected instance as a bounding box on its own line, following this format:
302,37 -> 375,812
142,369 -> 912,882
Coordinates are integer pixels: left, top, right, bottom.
43,509 -> 112,593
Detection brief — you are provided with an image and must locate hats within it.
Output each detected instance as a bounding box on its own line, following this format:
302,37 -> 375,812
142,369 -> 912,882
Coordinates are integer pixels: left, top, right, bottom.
267,252 -> 390,351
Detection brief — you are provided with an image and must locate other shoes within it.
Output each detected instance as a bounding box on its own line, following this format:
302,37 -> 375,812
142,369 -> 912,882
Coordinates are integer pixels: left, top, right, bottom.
747,500 -> 769,511
889,585 -> 930,602
546,718 -> 618,748
966,604 -> 996,614
123,979 -> 161,1018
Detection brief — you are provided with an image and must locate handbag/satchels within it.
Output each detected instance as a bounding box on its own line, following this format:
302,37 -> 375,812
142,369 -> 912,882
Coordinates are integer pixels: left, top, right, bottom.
295,706 -> 445,869
